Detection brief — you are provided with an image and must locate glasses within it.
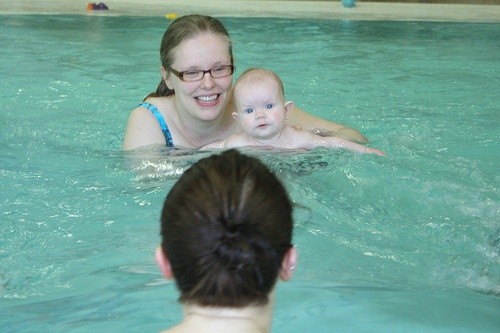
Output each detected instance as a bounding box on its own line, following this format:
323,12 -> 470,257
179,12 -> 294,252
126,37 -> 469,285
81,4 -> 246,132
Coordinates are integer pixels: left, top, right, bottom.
168,64 -> 234,82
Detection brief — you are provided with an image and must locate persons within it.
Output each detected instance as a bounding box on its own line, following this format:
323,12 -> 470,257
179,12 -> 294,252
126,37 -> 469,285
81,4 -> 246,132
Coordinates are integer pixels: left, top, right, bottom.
122,14 -> 363,152
155,148 -> 296,333
225,68 -> 366,151
